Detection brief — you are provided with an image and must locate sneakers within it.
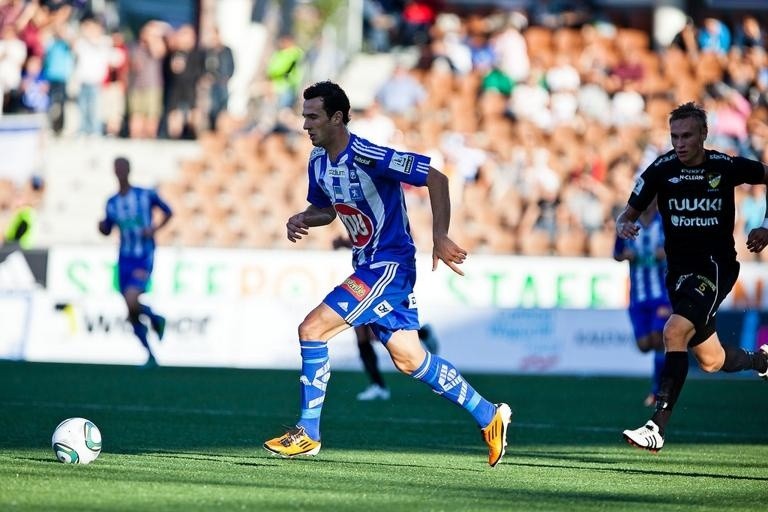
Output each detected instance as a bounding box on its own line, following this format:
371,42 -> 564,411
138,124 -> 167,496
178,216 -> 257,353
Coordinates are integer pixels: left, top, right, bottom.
622,419 -> 664,453
758,344 -> 768,381
421,324 -> 440,355
262,425 -> 322,459
481,403 -> 512,467
356,383 -> 391,400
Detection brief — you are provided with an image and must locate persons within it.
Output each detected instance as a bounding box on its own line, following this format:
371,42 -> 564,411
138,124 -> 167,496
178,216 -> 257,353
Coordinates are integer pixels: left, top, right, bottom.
98,156 -> 173,367
613,100 -> 768,450
262,80 -> 515,468
0,0 -> 235,138
614,197 -> 672,408
144,0 -> 768,256
0,175 -> 47,288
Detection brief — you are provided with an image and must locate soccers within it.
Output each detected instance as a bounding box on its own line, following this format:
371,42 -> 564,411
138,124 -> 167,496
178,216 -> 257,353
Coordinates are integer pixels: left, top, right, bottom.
52,417 -> 102,465
